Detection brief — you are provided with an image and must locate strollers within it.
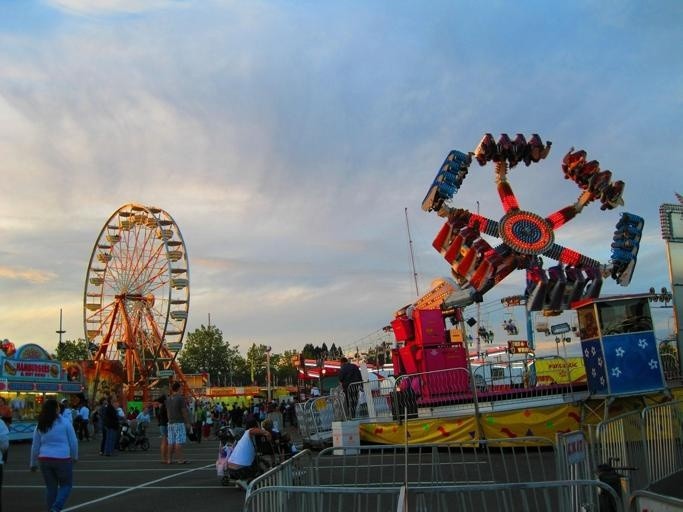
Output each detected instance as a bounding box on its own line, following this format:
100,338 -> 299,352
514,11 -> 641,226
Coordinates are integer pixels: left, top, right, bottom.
216,423 -> 246,486
114,416 -> 151,453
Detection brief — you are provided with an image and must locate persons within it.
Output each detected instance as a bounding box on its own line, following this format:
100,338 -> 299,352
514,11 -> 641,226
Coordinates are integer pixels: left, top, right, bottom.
262,402 -> 294,464
338,355 -> 364,421
460,318 -> 518,347
58,381 -> 191,464
185,399 -> 293,444
29,398 -> 80,511
0,419 -> 10,511
227,419 -> 273,491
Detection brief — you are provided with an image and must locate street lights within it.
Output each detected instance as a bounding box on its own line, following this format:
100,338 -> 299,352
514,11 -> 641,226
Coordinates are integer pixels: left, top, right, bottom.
550,320 -> 579,406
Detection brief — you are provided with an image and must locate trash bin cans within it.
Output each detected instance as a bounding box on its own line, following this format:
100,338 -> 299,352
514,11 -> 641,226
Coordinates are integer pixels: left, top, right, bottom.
268,412 -> 283,433
